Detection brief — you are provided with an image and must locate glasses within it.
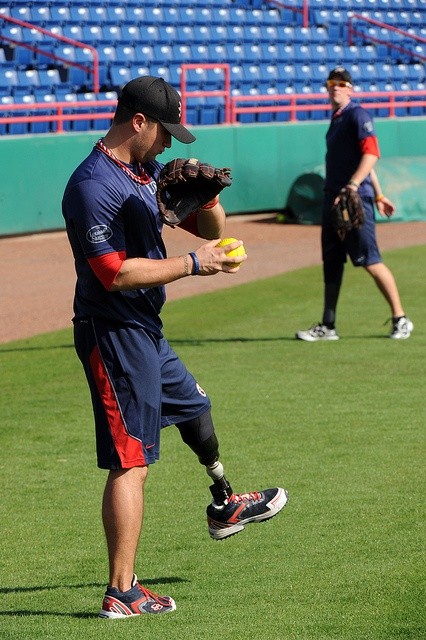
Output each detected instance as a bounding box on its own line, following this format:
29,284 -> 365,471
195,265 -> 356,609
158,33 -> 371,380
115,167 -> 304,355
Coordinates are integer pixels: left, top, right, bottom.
327,79 -> 352,88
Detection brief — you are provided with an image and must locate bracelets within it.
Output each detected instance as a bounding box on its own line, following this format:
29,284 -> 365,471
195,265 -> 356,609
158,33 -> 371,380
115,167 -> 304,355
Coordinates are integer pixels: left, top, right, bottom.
345,181 -> 359,192
189,252 -> 200,274
375,195 -> 385,203
201,199 -> 219,209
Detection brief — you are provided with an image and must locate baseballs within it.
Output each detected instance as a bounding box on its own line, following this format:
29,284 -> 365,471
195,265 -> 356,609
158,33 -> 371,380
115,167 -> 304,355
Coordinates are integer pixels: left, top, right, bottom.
217,238 -> 245,269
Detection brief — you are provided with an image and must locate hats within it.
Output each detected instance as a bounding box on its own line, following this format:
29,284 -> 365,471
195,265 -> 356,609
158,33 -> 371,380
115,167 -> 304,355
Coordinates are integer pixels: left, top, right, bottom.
117,76 -> 197,144
328,68 -> 352,85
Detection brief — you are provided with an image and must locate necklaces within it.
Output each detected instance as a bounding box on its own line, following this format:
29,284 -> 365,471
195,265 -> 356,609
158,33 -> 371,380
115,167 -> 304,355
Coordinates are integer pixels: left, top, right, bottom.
332,99 -> 351,117
96,138 -> 152,186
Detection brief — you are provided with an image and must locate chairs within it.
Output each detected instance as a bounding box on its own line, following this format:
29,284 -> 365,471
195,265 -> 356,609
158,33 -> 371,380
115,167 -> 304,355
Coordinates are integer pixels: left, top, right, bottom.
67,63 -> 425,92
0,24 -> 332,45
180,93 -> 426,125
337,27 -> 426,41
285,1 -> 426,9
297,12 -> 426,26
0,1 -> 286,24
10,42 -> 382,66
392,42 -> 426,60
1,64 -> 71,96
1,92 -> 119,136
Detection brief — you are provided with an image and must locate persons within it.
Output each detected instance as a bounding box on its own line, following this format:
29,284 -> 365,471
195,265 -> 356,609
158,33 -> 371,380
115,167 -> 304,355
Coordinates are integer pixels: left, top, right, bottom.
61,75 -> 288,619
294,65 -> 413,342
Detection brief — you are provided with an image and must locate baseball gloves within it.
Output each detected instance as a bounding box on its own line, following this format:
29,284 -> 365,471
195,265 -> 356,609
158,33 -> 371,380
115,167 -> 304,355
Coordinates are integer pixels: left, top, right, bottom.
157,158 -> 232,228
333,187 -> 365,228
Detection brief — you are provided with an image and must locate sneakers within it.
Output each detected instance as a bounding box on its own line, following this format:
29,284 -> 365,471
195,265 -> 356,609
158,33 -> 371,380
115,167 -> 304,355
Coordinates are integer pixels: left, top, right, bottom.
381,316 -> 414,340
206,488 -> 288,540
296,324 -> 339,341
99,573 -> 176,618
